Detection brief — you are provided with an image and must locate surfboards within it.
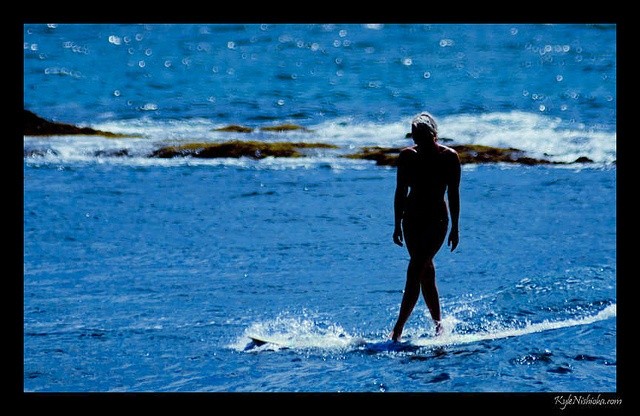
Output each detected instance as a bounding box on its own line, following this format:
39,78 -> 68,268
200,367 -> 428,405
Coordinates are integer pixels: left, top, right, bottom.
246,316 -> 607,349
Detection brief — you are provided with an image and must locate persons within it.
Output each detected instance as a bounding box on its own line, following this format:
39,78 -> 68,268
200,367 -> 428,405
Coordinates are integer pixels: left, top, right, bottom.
389,111 -> 462,344
